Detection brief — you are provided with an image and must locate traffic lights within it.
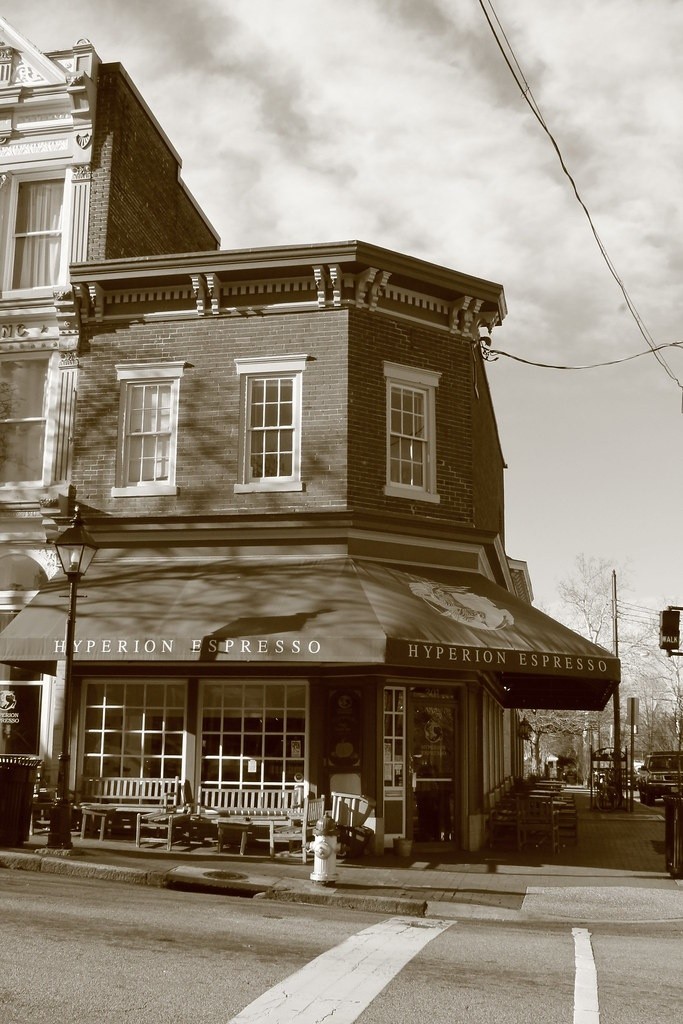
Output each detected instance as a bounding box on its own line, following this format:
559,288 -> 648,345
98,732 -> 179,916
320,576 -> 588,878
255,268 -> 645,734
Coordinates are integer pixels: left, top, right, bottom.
657,609 -> 680,652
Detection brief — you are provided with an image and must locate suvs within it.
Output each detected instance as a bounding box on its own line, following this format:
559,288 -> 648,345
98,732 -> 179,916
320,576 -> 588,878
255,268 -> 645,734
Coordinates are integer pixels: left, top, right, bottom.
636,755 -> 683,807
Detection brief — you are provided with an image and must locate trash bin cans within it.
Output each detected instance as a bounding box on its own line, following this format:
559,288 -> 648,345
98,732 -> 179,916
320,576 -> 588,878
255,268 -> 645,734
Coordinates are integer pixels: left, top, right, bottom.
0,753 -> 44,849
661,793 -> 683,879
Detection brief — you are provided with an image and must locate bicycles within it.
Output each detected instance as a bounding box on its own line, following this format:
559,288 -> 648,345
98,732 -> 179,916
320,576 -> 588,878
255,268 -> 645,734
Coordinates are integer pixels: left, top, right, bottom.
595,770 -> 624,814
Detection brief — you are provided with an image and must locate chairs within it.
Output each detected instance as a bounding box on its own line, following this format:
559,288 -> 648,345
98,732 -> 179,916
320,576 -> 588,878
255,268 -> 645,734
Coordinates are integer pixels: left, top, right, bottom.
516,795 -> 560,856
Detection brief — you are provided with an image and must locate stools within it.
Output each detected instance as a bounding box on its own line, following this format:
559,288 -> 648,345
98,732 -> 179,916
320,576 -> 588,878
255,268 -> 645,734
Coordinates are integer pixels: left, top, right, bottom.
214,819 -> 256,856
136,810 -> 191,852
80,805 -> 117,842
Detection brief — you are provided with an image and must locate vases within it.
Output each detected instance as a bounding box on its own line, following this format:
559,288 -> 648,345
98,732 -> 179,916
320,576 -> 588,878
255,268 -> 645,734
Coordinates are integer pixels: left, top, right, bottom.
394,838 -> 412,857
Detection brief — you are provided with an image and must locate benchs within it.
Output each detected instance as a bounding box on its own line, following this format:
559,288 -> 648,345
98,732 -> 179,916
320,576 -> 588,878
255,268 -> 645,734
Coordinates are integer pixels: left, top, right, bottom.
270,794 -> 327,863
187,780 -> 311,852
73,775 -> 180,836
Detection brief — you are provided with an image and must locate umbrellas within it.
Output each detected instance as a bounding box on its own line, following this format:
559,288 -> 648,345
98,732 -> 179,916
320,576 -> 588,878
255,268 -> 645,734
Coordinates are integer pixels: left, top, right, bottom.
593,747 -> 626,767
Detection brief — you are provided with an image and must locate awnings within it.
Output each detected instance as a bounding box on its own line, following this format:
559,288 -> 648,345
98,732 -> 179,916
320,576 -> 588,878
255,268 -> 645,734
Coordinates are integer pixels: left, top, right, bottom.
0,557 -> 622,711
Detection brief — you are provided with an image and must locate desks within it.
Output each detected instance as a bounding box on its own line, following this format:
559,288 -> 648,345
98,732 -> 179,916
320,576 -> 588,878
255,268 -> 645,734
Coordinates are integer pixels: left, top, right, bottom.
487,780 -> 567,844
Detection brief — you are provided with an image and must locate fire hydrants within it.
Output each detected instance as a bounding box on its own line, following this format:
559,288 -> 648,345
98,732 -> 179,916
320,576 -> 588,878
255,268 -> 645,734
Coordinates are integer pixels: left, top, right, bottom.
300,811 -> 349,888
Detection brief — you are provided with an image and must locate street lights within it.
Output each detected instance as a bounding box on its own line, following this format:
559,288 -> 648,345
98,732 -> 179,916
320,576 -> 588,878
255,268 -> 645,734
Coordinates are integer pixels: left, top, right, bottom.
44,501 -> 102,851
